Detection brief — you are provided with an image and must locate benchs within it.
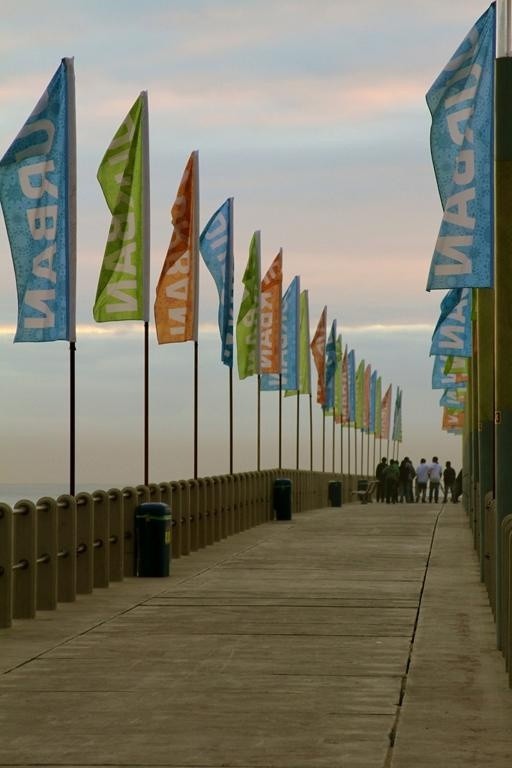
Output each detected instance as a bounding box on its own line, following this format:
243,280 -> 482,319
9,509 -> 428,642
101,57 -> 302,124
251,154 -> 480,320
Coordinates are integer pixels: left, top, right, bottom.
351,480 -> 381,506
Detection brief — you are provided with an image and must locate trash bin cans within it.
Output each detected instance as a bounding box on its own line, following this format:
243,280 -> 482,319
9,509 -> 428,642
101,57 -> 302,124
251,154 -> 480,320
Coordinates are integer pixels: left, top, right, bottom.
358,479 -> 368,500
134,502 -> 174,577
273,479 -> 292,520
328,480 -> 344,507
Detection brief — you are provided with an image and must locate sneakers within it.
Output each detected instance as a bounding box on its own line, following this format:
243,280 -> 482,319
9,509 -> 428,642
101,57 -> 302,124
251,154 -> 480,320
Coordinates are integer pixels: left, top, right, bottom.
376,496 -> 460,505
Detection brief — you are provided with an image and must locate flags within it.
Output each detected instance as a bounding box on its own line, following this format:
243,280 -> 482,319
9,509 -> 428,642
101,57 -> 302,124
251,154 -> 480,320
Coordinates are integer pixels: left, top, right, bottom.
421,0 -> 511,291
238,231 -> 404,444
0,57 -> 75,346
199,199 -> 235,368
152,152 -> 200,342
92,89 -> 152,323
428,287 -> 472,435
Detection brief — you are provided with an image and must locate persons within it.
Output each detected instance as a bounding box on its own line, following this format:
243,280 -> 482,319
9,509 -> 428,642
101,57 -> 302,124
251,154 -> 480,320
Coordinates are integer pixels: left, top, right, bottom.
442,461 -> 456,503
428,457 -> 442,504
415,458 -> 429,503
374,456 -> 415,505
451,468 -> 463,504
